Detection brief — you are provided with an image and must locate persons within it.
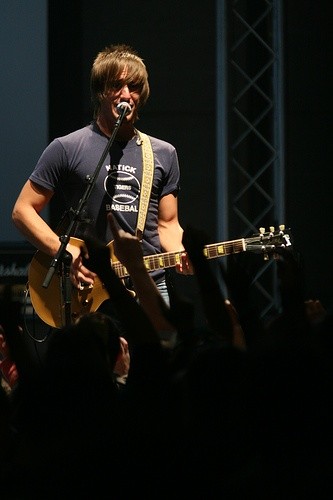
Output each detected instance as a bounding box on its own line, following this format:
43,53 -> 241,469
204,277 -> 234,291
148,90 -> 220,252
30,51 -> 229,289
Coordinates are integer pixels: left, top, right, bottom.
11,44 -> 195,332
0,297 -> 333,500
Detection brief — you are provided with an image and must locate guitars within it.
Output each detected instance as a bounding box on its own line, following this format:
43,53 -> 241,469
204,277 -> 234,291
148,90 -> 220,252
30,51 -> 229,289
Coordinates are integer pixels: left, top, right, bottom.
26,223 -> 293,330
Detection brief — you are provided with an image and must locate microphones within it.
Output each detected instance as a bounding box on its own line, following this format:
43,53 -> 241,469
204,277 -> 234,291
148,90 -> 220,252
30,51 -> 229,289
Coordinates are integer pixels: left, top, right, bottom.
116,102 -> 132,116
